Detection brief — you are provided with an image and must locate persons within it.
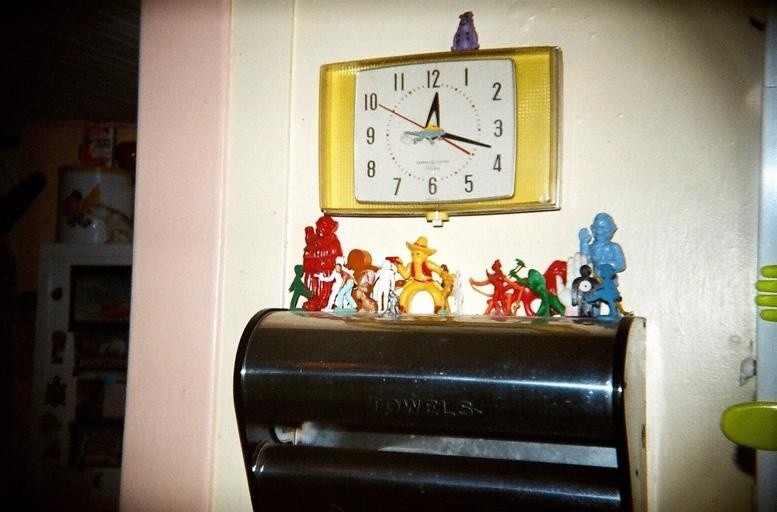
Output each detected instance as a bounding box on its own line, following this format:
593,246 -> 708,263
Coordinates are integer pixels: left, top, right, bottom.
335,263 -> 358,309
289,265 -> 314,309
578,213 -> 626,317
572,265 -> 600,316
393,236 -> 454,314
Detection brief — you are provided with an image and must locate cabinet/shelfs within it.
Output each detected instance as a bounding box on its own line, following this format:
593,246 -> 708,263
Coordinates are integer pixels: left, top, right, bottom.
26,242 -> 134,512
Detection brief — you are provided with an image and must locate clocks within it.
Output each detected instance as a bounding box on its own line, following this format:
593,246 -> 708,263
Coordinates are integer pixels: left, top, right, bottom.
319,46 -> 562,226
